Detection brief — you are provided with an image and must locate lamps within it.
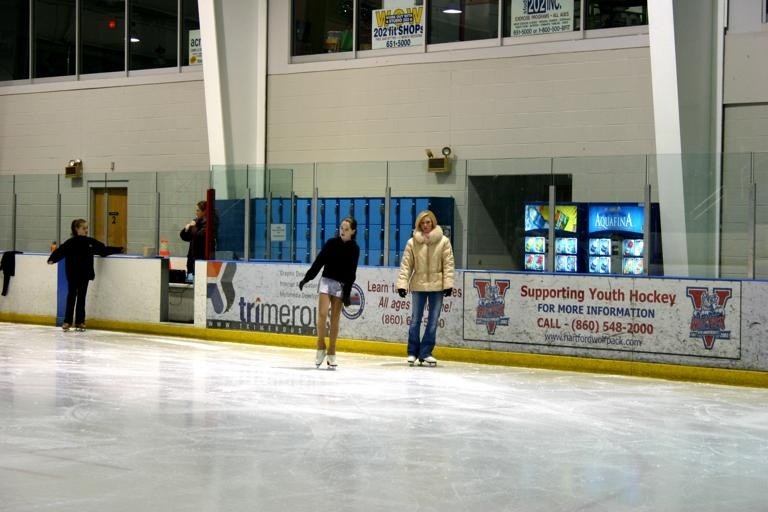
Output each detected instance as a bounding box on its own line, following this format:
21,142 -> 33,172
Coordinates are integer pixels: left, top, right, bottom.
65,157 -> 82,179
424,146 -> 453,173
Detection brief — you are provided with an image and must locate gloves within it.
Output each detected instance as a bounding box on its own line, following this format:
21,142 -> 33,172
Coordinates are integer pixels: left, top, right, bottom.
442,289 -> 452,297
299,280 -> 305,290
398,289 -> 406,297
344,295 -> 351,306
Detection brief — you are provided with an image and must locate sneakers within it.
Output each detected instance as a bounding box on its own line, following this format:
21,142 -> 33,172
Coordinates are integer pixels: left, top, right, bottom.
316,349 -> 325,365
75,323 -> 86,328
62,323 -> 71,329
419,356 -> 436,363
408,355 -> 416,362
327,355 -> 337,366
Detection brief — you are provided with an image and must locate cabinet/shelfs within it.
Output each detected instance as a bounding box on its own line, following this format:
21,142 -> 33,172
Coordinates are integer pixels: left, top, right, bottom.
254,196 -> 455,268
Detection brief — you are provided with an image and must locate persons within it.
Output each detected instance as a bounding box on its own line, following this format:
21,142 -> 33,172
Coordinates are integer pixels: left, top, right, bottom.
47,219 -> 123,330
298,217 -> 360,366
397,203 -> 455,362
180,201 -> 219,282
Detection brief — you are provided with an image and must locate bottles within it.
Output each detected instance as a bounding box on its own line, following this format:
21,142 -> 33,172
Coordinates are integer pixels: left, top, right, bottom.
158,239 -> 169,256
623,240 -> 643,275
527,205 -> 574,233
50,241 -> 57,253
589,239 -> 609,273
